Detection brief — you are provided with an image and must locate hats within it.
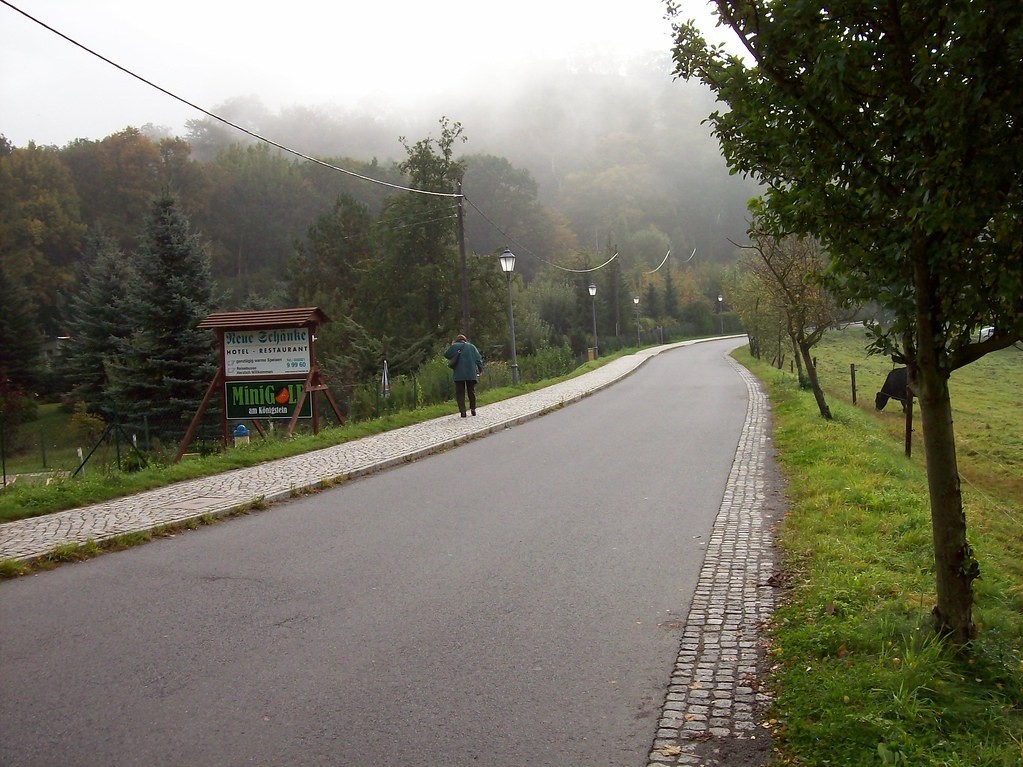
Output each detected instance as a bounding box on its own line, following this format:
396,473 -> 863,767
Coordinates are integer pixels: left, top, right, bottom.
455,335 -> 466,341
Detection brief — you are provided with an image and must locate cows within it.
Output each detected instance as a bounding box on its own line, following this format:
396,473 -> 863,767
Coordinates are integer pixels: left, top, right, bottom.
875,367 -> 918,414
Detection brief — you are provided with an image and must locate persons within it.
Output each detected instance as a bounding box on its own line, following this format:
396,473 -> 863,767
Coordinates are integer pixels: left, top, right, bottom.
445,335 -> 482,418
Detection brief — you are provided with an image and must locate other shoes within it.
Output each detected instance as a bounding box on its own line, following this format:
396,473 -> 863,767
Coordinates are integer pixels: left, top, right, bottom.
461,412 -> 465,417
471,409 -> 476,416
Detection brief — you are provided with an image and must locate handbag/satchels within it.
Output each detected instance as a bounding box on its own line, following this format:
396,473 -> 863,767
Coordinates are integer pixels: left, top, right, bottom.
448,349 -> 461,368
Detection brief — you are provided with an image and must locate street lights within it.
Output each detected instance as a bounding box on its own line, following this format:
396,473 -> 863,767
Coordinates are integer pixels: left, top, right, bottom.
587,281 -> 599,360
717,293 -> 723,335
633,294 -> 640,347
496,242 -> 519,388
